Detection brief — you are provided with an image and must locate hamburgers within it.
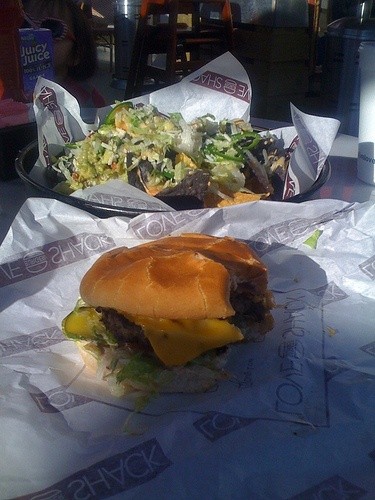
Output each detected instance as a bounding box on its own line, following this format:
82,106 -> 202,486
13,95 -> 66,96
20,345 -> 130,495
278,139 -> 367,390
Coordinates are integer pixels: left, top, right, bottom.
61,233 -> 274,400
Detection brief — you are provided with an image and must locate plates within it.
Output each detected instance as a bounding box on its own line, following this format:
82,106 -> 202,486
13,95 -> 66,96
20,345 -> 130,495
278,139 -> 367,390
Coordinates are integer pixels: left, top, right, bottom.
14,124 -> 332,219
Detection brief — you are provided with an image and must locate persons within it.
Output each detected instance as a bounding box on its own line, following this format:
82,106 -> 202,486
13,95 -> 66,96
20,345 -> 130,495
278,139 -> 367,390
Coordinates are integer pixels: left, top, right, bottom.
0,0 -> 107,130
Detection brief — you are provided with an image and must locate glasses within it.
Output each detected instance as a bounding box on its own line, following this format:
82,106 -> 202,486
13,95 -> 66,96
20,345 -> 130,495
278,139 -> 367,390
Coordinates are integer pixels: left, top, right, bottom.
17,14 -> 76,42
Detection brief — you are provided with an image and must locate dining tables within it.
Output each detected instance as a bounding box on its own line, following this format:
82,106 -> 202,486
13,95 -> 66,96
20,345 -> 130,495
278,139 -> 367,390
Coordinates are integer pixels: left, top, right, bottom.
0,116 -> 375,500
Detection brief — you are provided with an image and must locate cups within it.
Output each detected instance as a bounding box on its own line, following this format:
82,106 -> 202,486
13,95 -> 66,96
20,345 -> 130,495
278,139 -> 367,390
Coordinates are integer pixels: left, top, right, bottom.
355,38 -> 375,187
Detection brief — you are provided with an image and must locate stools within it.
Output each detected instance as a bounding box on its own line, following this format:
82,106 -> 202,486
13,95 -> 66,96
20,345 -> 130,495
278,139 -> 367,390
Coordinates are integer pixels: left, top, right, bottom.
125,0 -> 235,101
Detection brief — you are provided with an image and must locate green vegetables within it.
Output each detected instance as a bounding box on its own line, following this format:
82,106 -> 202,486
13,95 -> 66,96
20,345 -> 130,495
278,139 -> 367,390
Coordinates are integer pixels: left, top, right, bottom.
50,101 -> 263,191
